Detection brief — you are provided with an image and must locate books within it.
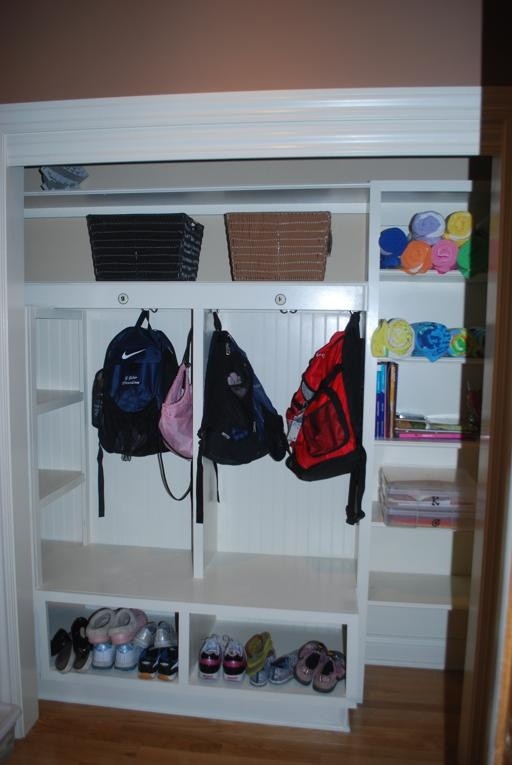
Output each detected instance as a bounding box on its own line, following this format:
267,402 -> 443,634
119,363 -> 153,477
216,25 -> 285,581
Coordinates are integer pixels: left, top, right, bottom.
83,206 -> 329,278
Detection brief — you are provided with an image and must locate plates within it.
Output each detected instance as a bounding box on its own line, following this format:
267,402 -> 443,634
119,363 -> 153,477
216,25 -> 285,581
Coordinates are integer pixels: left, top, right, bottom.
4,156 -> 486,737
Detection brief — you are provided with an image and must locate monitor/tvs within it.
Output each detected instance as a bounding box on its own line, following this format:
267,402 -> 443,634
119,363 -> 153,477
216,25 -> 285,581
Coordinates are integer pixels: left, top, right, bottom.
374,360 -> 485,442
380,477 -> 468,530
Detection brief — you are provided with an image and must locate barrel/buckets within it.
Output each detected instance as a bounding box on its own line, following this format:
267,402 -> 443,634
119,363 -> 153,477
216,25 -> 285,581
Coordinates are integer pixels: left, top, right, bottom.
242,631 -> 274,678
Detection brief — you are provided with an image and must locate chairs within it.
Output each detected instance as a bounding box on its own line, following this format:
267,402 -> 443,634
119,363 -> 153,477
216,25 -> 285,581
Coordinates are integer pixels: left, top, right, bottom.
248,639 -> 348,695
195,631 -> 249,684
47,605 -> 180,684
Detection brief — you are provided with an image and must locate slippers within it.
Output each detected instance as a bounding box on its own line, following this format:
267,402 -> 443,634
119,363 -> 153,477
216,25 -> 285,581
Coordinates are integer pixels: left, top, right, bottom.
197,309 -> 289,468
90,307 -> 194,464
285,311 -> 366,483
